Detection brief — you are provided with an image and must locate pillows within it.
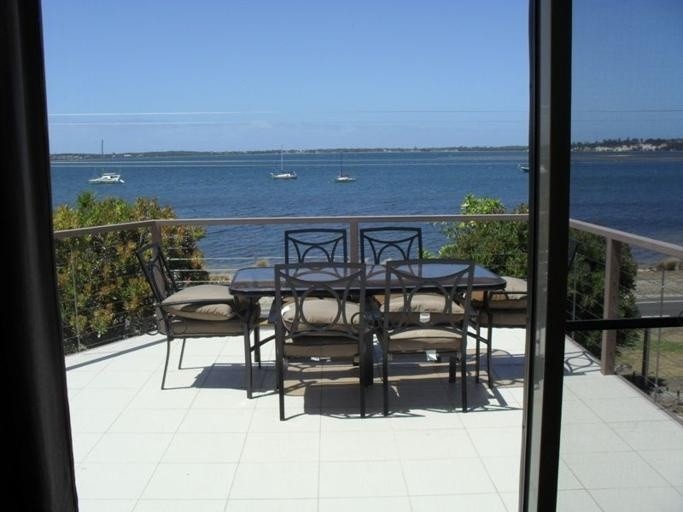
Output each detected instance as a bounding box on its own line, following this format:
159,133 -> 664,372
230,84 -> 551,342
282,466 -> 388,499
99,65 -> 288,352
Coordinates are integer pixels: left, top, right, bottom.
282,297 -> 361,335
463,276 -> 527,311
161,285 -> 262,321
373,293 -> 465,325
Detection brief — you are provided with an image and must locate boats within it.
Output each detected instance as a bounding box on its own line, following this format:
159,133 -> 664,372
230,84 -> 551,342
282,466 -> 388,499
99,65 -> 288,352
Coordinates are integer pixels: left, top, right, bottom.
520,163 -> 529,173
88,172 -> 125,185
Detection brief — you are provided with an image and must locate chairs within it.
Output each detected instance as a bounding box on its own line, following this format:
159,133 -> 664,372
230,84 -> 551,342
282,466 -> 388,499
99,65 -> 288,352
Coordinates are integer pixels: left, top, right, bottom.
285,228 -> 347,263
460,238 -> 580,389
274,262 -> 372,421
359,226 -> 422,323
372,258 -> 476,416
135,240 -> 261,398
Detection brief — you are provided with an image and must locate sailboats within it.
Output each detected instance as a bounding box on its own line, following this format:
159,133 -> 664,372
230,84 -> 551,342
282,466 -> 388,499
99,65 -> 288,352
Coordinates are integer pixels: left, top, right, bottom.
335,153 -> 357,183
269,147 -> 297,179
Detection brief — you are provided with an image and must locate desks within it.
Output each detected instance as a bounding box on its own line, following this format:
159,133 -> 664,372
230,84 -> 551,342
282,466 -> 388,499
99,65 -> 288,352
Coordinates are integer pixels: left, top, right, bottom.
229,263 -> 506,388
